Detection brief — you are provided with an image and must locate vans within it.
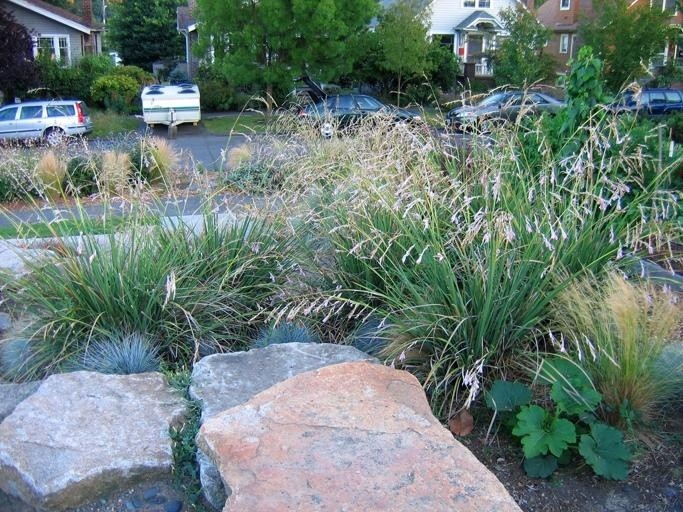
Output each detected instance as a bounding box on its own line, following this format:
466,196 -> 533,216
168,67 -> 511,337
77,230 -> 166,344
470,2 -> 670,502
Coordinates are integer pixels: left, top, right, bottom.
0,99 -> 93,148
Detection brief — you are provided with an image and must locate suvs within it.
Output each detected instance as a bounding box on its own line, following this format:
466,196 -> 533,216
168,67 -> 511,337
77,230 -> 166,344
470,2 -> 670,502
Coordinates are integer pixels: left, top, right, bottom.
603,88 -> 683,117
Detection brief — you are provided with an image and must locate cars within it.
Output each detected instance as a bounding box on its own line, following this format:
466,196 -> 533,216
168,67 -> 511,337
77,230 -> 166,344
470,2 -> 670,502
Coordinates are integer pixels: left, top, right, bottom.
292,72 -> 425,141
445,89 -> 576,135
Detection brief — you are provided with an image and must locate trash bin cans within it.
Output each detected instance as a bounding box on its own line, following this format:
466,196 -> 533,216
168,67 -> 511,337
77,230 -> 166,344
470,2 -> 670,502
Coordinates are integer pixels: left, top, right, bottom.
463,63 -> 476,77
